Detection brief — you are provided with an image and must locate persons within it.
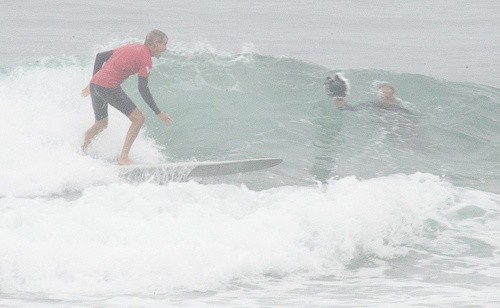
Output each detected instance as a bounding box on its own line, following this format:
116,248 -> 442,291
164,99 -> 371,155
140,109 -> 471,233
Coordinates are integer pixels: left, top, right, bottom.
81,29 -> 171,165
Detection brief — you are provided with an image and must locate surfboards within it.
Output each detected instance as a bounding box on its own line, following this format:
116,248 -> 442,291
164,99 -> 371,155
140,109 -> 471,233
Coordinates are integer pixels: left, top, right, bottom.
114,158 -> 283,176
